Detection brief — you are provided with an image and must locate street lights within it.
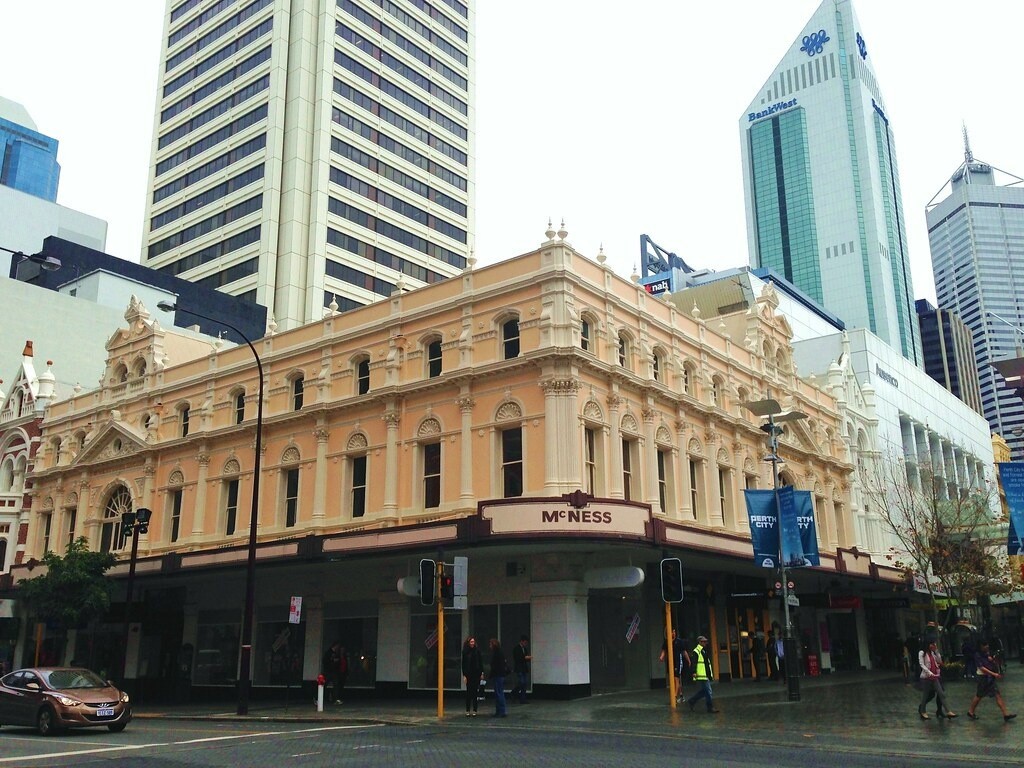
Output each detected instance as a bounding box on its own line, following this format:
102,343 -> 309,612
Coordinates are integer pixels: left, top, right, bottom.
155,300 -> 263,716
742,389 -> 821,700
114,507 -> 153,714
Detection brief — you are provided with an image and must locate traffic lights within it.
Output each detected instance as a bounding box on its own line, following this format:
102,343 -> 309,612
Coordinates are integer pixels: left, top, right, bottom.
658,558 -> 684,605
441,564 -> 454,599
420,559 -> 435,607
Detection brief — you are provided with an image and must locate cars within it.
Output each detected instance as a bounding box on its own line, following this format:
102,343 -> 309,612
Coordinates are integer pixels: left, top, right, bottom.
0,667 -> 131,737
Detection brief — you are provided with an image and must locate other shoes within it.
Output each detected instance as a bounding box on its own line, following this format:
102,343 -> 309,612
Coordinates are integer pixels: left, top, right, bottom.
507,694 -> 529,704
494,713 -> 505,719
688,703 -> 719,714
967,711 -> 978,720
466,710 -> 476,717
1004,714 -> 1016,721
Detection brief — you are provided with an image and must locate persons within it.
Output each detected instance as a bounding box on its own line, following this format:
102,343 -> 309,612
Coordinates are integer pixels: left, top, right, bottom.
891,628 -> 929,679
461,636 -> 484,716
685,635 -> 720,712
486,638 -> 508,718
508,636 -> 534,705
744,627 -> 788,681
966,639 -> 1018,722
336,644 -> 348,705
917,638 -> 959,721
922,636 -> 948,718
657,625 -> 691,708
321,640 -> 344,707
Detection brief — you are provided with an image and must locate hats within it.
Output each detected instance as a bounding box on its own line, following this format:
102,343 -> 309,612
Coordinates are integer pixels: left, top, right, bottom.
697,635 -> 709,642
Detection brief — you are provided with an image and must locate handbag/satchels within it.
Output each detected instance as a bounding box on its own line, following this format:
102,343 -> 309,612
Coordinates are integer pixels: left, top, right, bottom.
478,680 -> 487,705
975,666 -> 989,678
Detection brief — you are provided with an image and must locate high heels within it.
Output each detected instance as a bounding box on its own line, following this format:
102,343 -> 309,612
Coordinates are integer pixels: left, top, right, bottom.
918,710 -> 931,720
936,710 -> 946,718
947,712 -> 958,719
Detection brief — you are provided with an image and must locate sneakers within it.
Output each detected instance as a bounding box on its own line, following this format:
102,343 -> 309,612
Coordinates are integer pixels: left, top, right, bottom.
676,697 -> 686,704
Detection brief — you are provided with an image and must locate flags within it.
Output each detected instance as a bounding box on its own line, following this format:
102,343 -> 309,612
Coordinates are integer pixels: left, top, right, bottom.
793,490 -> 821,567
743,489 -> 781,570
1007,515 -> 1024,557
777,485 -> 806,566
998,462 -> 1024,552
